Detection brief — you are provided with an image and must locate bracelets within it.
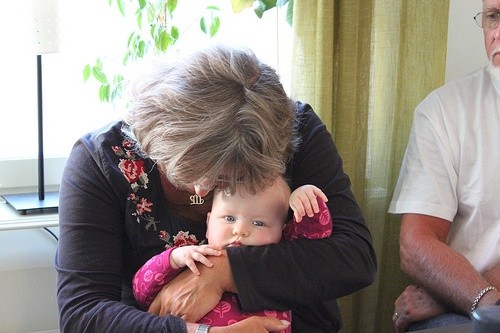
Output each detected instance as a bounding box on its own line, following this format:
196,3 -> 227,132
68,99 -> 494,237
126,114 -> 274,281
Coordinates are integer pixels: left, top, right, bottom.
469,287 -> 497,319
195,324 -> 212,333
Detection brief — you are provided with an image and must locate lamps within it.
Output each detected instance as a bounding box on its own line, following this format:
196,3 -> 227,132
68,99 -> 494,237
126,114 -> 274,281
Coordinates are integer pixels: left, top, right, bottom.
0,0 -> 64,216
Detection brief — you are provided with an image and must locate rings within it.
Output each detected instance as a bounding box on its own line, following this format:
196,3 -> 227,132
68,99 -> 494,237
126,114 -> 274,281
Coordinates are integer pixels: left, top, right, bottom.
395,312 -> 399,318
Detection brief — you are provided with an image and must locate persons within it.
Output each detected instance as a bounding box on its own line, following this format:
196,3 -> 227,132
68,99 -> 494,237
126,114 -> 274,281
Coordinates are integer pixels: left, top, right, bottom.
131,170 -> 332,333
53,45 -> 379,333
387,0 -> 500,333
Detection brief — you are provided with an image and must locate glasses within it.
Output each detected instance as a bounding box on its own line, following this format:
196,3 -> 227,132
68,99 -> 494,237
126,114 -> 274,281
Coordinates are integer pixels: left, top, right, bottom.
474,11 -> 500,29
